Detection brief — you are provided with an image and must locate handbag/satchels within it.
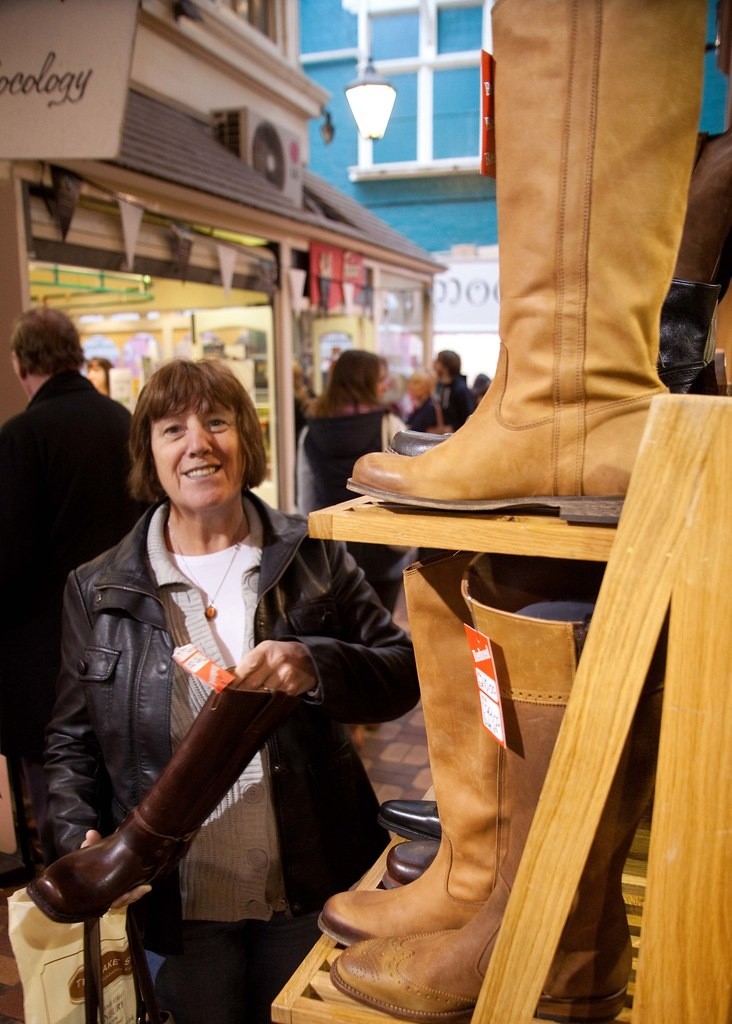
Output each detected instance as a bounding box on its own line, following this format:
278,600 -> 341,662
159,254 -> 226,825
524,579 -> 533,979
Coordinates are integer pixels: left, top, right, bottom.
427,401 -> 453,435
8,888 -> 136,1024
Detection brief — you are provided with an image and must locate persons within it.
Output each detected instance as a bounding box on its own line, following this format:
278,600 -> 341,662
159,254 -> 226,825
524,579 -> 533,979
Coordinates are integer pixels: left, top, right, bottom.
42,360 -> 420,1024
0,309 -> 154,868
294,371 -> 318,449
296,349 -> 418,750
405,351 -> 478,433
471,374 -> 491,397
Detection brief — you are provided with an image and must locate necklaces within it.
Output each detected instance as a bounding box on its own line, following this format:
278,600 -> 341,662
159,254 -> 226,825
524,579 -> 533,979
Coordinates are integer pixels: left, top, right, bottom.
175,517 -> 246,618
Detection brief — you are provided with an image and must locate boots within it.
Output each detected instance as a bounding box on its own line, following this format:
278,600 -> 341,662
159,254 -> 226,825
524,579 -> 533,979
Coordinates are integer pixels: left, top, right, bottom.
27,688 -> 325,923
331,553 -> 666,1023
317,551 -> 509,948
384,279 -> 721,458
345,0 -> 707,526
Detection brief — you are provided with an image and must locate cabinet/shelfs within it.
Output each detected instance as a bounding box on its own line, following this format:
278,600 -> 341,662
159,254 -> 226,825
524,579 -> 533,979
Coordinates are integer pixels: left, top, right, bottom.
270,393 -> 732,1024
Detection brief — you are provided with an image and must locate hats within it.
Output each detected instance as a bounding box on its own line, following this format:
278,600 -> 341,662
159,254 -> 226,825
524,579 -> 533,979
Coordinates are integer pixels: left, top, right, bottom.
472,374 -> 492,396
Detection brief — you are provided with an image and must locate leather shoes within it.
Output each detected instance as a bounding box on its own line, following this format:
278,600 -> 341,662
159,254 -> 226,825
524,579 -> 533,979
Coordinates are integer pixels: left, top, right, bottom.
382,840 -> 439,890
377,798 -> 441,841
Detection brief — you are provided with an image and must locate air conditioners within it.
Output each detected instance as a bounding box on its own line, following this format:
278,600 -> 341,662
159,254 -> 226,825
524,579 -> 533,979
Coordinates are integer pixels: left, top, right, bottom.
212,105 -> 304,207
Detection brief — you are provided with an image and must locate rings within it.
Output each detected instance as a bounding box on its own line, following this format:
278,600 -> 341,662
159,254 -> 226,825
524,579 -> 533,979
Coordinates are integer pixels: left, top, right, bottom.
262,684 -> 270,692
